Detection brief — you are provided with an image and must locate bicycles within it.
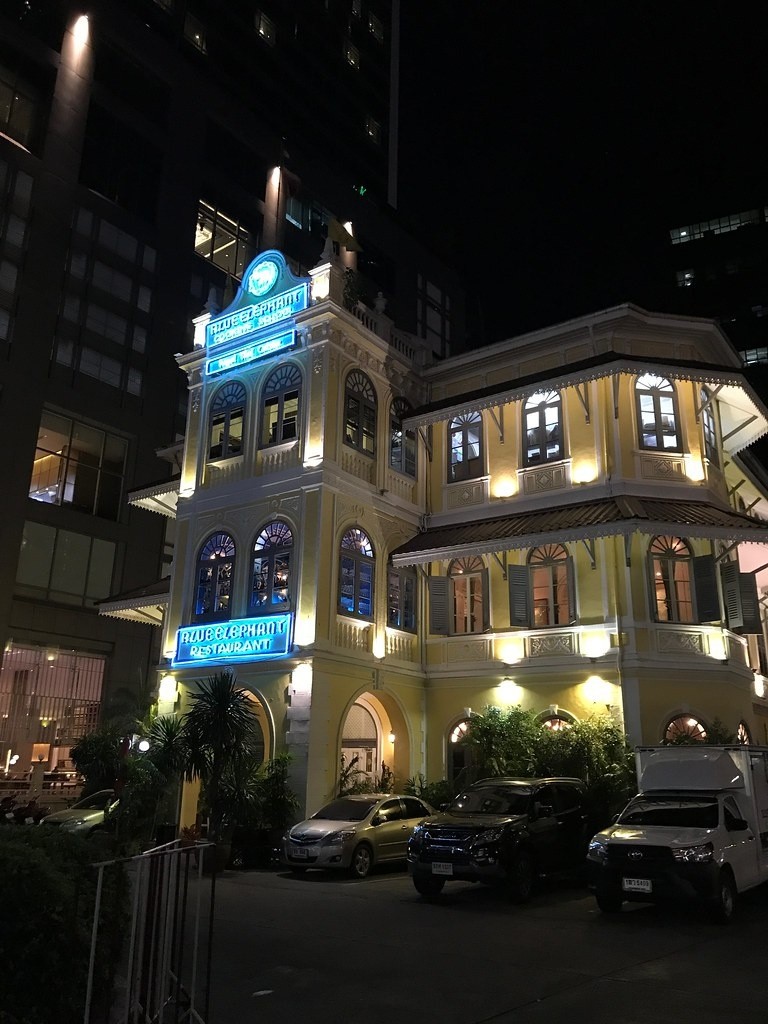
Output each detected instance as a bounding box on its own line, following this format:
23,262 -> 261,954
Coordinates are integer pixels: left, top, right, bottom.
61,796 -> 76,810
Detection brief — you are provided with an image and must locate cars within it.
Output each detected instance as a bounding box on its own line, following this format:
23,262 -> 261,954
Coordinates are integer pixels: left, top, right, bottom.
39,789 -> 120,849
283,794 -> 440,878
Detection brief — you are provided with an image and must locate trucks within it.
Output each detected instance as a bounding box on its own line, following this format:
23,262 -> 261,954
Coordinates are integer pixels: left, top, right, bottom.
584,746 -> 768,918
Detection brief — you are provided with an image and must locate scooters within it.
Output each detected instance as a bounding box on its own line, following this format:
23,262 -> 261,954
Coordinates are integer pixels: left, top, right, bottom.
0,794 -> 53,826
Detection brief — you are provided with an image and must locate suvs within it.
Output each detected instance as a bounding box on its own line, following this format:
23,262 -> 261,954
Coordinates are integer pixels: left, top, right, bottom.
406,778 -> 602,898
